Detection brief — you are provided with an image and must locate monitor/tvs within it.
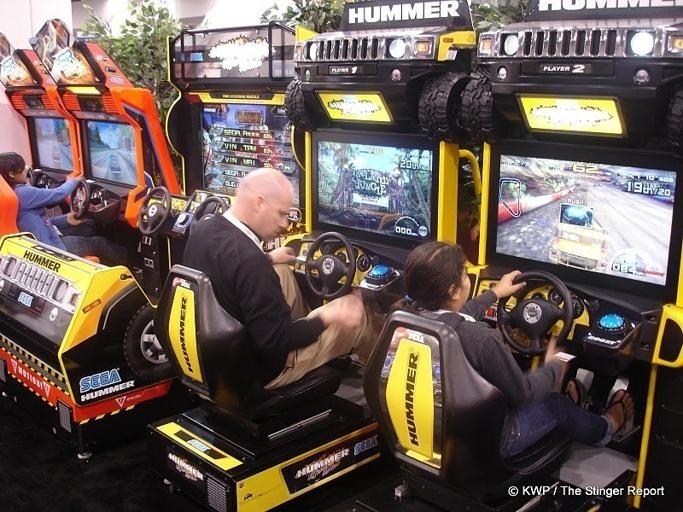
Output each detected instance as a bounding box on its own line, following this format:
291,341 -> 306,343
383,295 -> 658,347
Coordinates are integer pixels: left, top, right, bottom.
87,121 -> 138,187
34,118 -> 74,172
494,153 -> 677,287
316,140 -> 434,242
200,103 -> 300,209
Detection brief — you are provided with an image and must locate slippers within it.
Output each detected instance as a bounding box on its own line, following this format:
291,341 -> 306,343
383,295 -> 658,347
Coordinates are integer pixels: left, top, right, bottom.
603,389 -> 637,442
560,379 -> 587,407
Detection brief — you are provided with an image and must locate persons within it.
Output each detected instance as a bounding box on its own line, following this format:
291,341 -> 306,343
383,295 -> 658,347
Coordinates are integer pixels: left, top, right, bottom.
386,241 -> 636,459
0,152 -> 128,266
182,167 -> 379,390
